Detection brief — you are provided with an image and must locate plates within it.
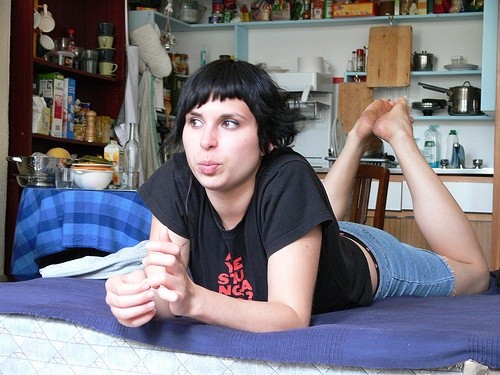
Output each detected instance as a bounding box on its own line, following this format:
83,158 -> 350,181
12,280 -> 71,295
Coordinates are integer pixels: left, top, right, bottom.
72,163 -> 113,167
443,63 -> 478,70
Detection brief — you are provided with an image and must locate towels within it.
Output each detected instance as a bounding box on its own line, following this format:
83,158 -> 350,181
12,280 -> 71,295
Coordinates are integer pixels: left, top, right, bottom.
129,23 -> 172,79
139,72 -> 162,184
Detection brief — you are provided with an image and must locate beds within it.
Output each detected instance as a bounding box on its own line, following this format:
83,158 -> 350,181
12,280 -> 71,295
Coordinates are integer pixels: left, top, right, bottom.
0,269 -> 500,375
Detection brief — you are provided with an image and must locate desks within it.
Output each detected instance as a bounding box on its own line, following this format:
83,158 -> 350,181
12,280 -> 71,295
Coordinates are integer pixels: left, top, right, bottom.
9,186 -> 152,281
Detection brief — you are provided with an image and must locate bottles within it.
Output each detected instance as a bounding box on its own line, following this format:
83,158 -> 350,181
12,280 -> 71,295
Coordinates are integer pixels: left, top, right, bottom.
346,44 -> 368,73
423,124 -> 440,168
199,45 -> 207,67
302,0 -> 311,19
174,53 -> 188,75
123,122 -> 140,190
446,129 -> 458,163
84,110 -> 97,142
68,28 -> 79,69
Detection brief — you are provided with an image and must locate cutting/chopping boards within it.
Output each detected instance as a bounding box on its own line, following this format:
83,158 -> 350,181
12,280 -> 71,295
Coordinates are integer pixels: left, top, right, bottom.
366,25 -> 413,88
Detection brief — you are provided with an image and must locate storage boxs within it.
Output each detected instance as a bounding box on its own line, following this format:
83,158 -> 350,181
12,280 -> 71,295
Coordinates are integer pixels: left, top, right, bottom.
35,72 -> 76,139
332,3 -> 377,17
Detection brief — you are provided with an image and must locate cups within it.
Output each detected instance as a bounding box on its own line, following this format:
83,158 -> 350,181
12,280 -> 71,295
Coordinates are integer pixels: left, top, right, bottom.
34,3 -> 55,57
410,51 -> 435,71
54,167 -> 73,189
81,23 -> 118,76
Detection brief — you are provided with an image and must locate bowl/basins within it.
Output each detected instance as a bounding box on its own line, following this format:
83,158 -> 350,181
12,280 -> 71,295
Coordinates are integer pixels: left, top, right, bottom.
51,51 -> 75,68
71,168 -> 114,189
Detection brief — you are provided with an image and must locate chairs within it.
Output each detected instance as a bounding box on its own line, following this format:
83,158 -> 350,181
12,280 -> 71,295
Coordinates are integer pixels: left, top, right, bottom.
343,164 -> 389,231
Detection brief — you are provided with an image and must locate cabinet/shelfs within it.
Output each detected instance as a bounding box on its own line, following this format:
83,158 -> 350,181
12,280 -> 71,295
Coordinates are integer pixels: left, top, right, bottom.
128,0 -> 499,272
4,0 -> 126,277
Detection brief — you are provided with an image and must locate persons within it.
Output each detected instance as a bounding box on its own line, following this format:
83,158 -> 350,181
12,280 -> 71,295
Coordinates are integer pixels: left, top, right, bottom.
106,58 -> 490,333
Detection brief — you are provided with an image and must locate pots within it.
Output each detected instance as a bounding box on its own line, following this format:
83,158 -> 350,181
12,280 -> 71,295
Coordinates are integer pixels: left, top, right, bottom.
6,154 -> 72,188
418,79 -> 484,116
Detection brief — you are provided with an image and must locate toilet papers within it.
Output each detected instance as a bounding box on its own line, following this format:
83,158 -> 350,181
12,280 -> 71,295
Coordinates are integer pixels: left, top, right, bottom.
297,56 -> 330,73
